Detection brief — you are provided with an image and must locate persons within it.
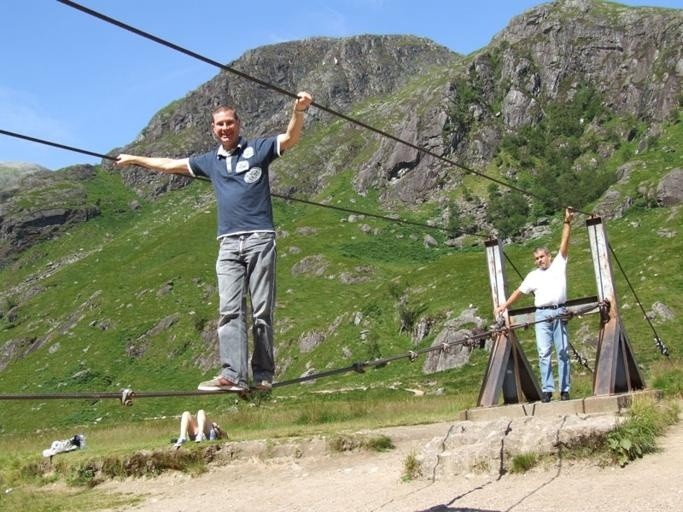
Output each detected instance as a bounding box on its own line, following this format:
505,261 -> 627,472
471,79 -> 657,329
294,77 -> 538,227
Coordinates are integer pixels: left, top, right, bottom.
114,90 -> 313,393
493,207 -> 577,402
173,410 -> 227,448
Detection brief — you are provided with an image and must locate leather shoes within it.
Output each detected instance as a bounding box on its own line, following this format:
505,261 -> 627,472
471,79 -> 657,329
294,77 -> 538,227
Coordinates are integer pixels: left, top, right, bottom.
560,391 -> 570,401
541,392 -> 552,403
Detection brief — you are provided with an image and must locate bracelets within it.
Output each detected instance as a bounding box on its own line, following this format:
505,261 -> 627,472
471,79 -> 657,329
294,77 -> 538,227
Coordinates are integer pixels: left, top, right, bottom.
216,424 -> 219,429
291,109 -> 305,114
563,222 -> 570,224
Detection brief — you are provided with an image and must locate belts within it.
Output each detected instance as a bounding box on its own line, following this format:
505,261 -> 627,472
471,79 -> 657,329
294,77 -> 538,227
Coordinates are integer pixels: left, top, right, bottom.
534,303 -> 567,311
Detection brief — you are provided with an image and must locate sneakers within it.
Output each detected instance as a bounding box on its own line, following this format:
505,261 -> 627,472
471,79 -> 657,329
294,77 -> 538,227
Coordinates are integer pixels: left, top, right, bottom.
172,436 -> 202,448
252,378 -> 274,393
196,375 -> 247,396
